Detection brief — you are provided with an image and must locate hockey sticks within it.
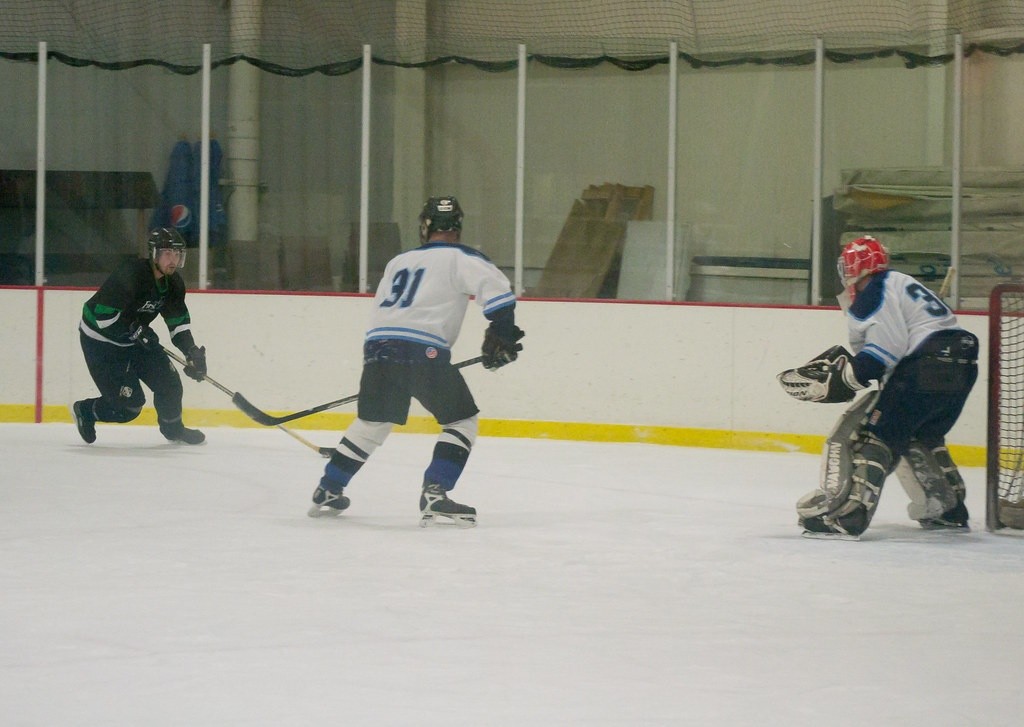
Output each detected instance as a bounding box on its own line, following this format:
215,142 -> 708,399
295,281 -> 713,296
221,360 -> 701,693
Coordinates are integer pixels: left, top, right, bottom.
230,342 -> 524,427
158,342 -> 337,458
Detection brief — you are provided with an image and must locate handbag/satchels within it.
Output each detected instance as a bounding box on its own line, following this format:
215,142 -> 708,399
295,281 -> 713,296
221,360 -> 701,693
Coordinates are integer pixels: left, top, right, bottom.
183,346 -> 208,383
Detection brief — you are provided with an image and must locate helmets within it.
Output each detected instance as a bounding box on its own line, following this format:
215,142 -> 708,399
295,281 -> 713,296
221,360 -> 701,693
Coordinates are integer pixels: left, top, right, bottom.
836,238 -> 888,302
421,195 -> 461,231
147,227 -> 184,259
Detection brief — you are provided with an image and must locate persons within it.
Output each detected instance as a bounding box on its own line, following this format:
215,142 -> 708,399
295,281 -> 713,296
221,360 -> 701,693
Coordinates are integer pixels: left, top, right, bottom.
73,221 -> 207,444
796,235 -> 980,543
307,195 -> 521,529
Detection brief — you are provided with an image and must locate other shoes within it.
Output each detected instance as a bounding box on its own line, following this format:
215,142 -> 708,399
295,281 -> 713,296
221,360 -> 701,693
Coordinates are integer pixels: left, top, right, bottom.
804,507 -> 868,536
942,503 -> 969,523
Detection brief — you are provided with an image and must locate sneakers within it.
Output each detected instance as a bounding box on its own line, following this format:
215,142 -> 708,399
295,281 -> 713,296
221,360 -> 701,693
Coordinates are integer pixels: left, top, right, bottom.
420,484 -> 477,529
166,427 -> 206,445
73,400 -> 97,444
308,483 -> 349,519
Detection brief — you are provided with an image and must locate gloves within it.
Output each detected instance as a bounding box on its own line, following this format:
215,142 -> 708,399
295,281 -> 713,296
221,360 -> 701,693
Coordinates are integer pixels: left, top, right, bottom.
129,325 -> 162,361
481,320 -> 525,368
